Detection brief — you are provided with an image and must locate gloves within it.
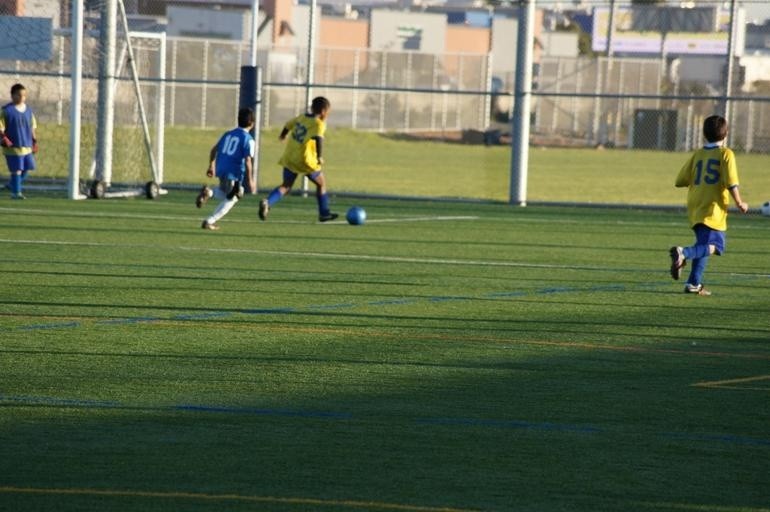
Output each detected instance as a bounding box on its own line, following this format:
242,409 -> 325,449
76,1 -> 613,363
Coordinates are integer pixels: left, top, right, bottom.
1,134 -> 13,148
31,138 -> 38,153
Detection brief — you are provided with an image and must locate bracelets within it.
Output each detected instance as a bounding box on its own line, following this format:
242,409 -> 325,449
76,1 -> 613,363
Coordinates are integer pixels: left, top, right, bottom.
32,138 -> 37,145
0,133 -> 6,138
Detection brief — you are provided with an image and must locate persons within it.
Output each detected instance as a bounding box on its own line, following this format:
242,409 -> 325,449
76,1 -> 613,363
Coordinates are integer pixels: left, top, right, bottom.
256,95 -> 338,222
0,82 -> 38,199
195,106 -> 257,230
669,114 -> 750,296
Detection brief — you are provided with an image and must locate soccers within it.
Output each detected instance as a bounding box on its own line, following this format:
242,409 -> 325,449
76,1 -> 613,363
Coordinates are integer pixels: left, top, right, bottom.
347,205 -> 367,223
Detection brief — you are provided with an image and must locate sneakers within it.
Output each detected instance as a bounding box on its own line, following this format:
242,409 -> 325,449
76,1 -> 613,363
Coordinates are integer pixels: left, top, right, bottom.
318,210 -> 338,221
201,218 -> 222,232
258,198 -> 271,221
669,245 -> 685,281
196,185 -> 212,208
11,191 -> 28,201
684,283 -> 712,297
